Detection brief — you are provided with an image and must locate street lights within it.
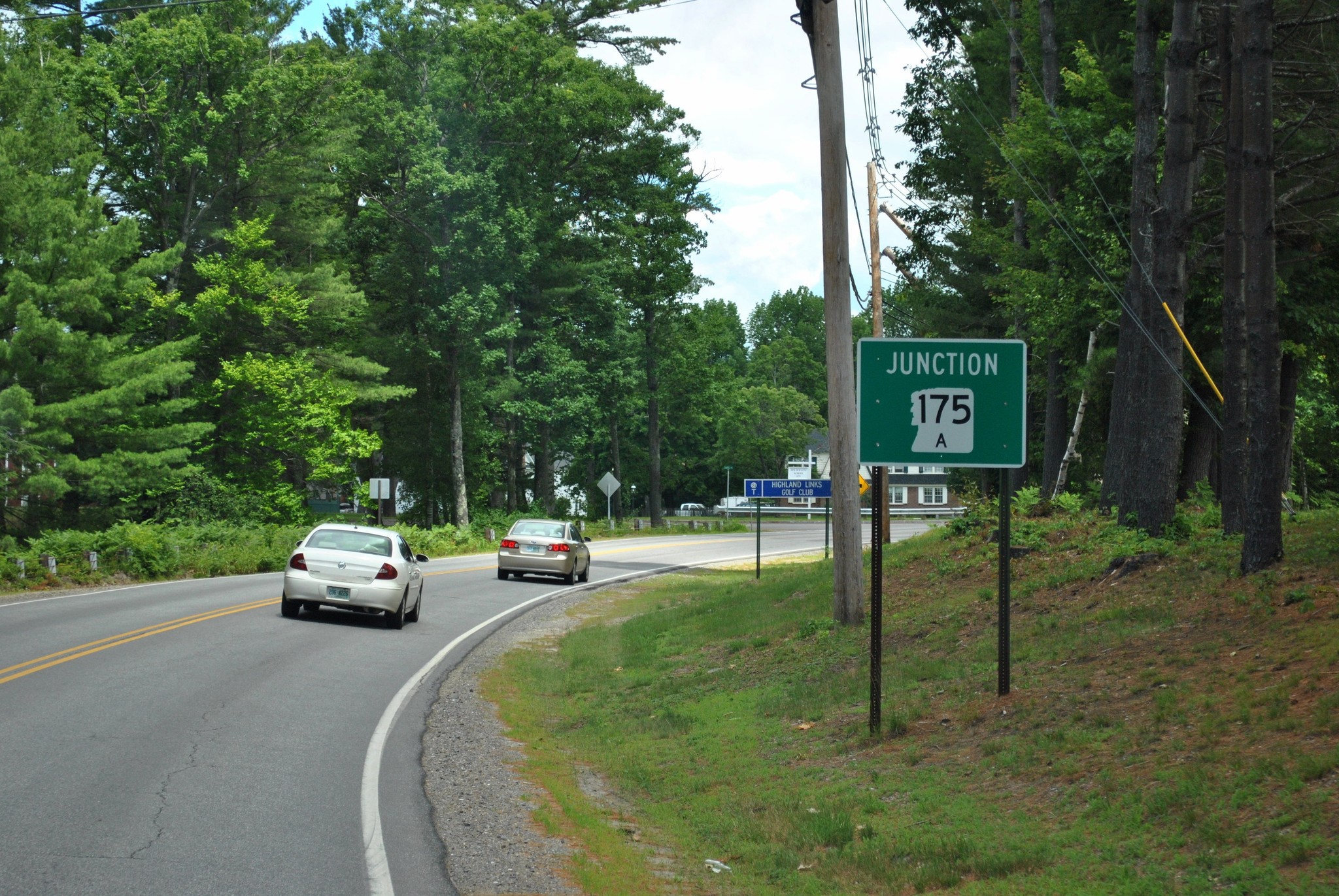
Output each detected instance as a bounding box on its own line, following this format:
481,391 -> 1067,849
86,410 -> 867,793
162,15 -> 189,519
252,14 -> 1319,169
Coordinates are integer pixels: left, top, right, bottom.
724,467 -> 733,521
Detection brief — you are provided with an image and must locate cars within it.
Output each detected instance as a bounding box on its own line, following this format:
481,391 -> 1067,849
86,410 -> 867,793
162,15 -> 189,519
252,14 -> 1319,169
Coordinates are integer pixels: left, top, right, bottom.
736,503 -> 758,507
680,503 -> 706,511
497,519 -> 591,586
281,523 -> 428,630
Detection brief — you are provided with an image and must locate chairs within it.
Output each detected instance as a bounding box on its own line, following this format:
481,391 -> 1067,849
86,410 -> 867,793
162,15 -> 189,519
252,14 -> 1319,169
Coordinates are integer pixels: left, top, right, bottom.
521,532 -> 563,538
319,541 -> 386,554
